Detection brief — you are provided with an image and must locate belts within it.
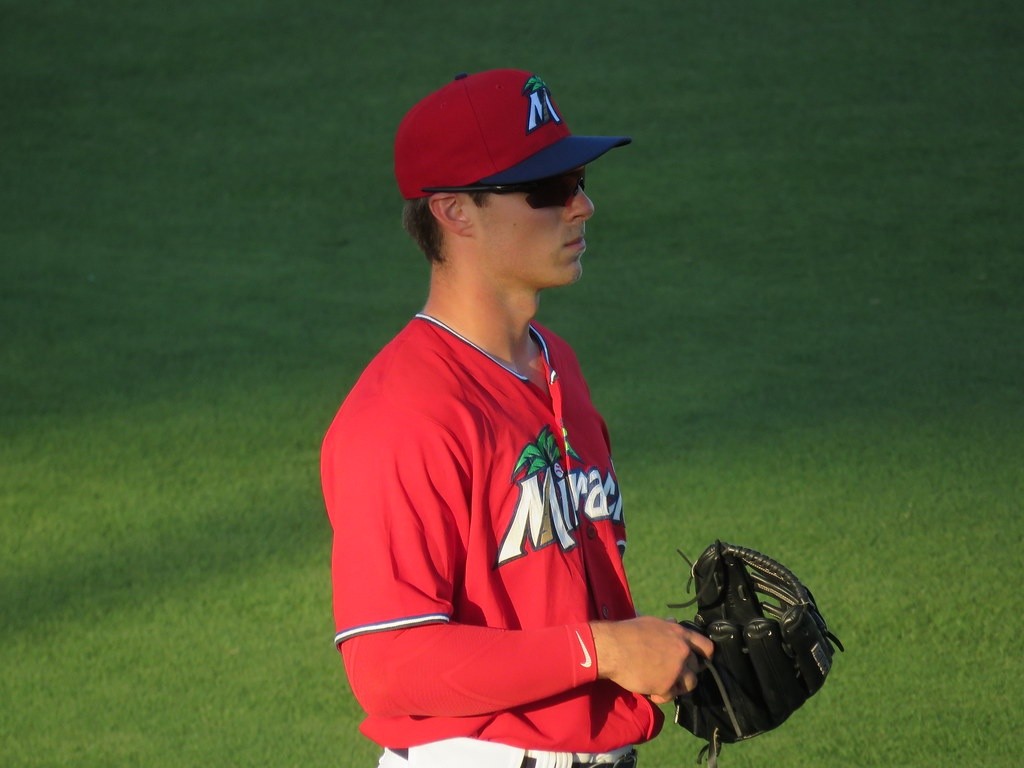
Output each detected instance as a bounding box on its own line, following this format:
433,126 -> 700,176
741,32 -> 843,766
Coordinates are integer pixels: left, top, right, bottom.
389,737 -> 638,768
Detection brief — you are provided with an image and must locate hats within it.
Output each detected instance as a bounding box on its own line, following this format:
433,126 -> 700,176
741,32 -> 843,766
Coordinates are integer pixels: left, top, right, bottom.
393,69 -> 633,199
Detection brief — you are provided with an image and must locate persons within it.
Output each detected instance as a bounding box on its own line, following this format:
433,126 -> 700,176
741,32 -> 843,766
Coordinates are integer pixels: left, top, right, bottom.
320,67 -> 715,768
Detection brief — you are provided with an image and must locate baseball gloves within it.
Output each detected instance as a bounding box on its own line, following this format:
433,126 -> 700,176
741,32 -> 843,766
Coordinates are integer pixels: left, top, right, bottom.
671,539 -> 837,745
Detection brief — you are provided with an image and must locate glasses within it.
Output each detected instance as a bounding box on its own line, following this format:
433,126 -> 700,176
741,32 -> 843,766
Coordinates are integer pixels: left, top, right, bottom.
422,167 -> 586,209
